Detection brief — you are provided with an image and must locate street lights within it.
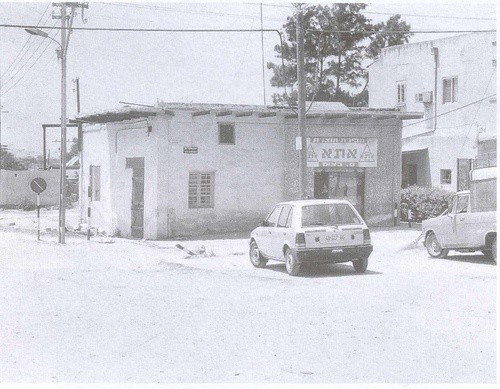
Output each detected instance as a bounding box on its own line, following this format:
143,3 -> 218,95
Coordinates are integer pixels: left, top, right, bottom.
23,27 -> 68,246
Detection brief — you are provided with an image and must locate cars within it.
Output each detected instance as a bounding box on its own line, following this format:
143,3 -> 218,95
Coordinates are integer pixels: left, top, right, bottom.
244,199 -> 374,277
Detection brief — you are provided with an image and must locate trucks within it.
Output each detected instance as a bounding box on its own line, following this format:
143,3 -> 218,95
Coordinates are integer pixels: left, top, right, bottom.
419,167 -> 497,267
